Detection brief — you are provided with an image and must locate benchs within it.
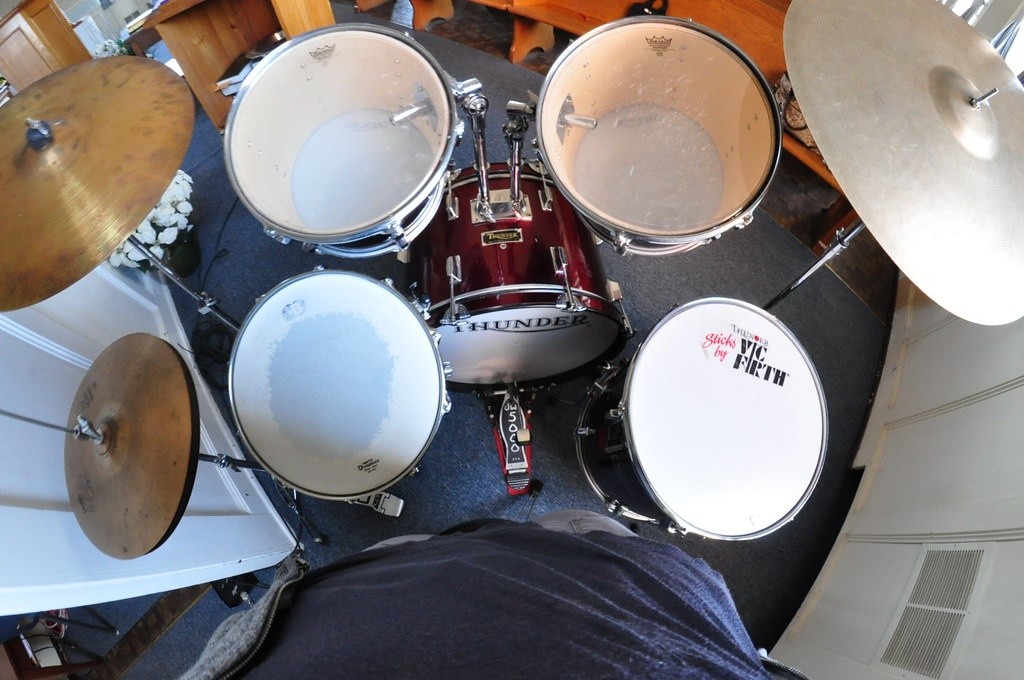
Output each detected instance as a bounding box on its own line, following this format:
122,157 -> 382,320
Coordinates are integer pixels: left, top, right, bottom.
352,0 -> 844,194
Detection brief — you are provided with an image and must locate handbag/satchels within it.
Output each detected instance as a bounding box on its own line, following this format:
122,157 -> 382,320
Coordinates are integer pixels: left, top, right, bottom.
625,0 -> 668,17
772,69 -> 821,155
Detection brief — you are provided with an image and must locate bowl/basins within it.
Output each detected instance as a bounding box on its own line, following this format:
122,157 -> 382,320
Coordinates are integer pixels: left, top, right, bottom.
245,29 -> 287,58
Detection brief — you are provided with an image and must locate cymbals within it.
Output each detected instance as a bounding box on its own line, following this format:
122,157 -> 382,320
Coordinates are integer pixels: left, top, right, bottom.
781,0 -> 1024,330
0,57 -> 196,315
63,332 -> 202,560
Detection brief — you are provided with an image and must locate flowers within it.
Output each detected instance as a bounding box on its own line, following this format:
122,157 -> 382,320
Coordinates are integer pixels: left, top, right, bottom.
111,168 -> 199,278
95,40 -> 156,59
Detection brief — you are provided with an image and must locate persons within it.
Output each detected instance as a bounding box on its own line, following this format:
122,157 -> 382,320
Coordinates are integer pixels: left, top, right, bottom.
174,511 -> 809,680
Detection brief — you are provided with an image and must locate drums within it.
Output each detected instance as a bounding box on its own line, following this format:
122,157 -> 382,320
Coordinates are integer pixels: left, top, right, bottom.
226,268 -> 445,503
406,160 -> 627,389
221,20 -> 458,260
574,294 -> 831,543
537,13 -> 782,257
